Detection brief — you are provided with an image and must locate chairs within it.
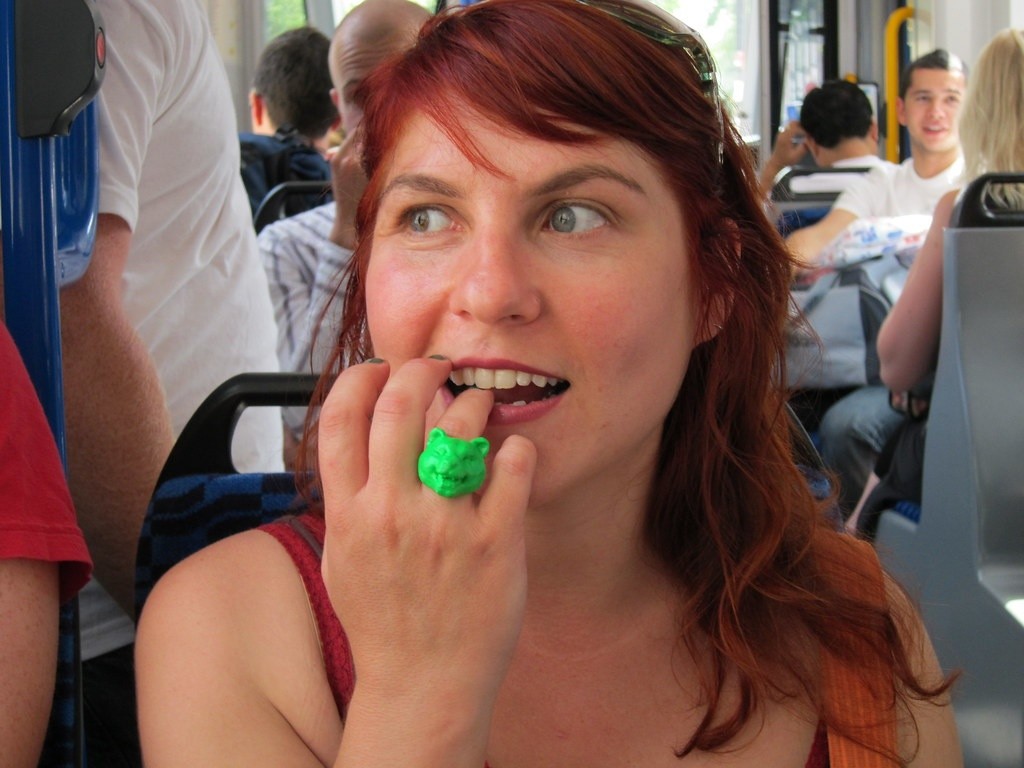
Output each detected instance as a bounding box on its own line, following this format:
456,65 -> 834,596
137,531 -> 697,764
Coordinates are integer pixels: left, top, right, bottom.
0,0 -> 332,768
763,160 -> 1024,767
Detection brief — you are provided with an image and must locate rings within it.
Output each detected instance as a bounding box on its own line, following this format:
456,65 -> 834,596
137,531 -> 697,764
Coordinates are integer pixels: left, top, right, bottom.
416,426 -> 490,499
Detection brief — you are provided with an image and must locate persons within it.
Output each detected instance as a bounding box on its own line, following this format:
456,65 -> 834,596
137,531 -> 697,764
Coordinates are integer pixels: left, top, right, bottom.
0,0 -> 1023,768
